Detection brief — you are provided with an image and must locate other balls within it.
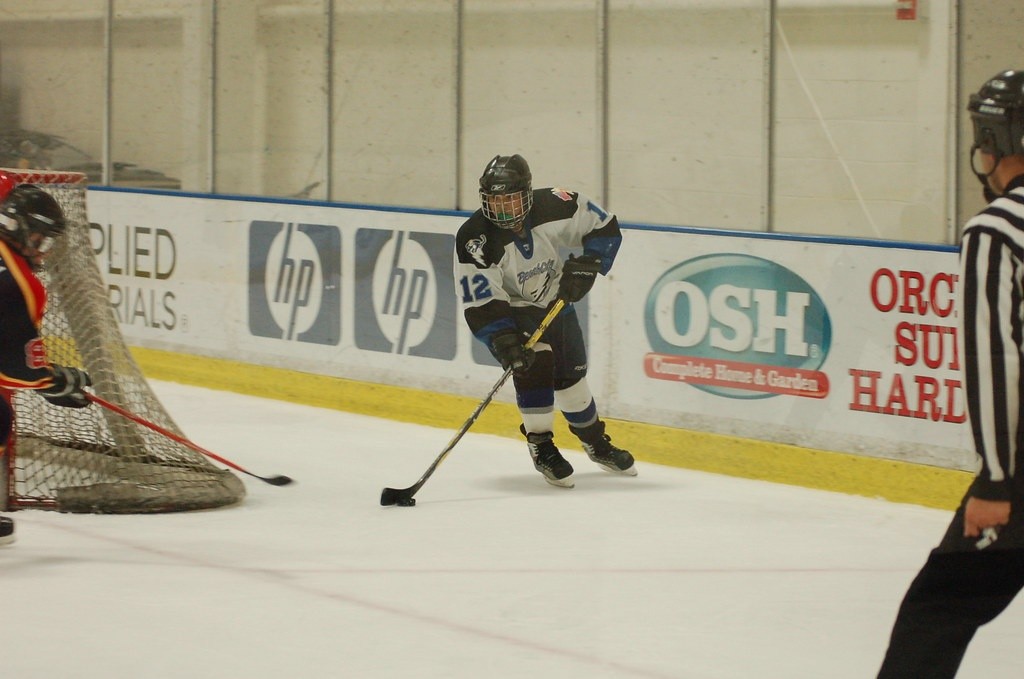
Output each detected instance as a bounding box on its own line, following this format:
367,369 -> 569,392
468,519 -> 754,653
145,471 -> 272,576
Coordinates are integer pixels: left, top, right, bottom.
397,498 -> 416,507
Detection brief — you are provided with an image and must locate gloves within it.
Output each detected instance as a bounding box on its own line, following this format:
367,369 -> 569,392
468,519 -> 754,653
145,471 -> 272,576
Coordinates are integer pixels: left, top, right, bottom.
34,363 -> 93,407
488,327 -> 537,377
556,250 -> 602,303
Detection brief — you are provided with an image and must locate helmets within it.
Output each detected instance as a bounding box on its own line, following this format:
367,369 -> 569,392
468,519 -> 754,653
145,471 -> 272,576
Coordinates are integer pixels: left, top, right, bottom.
479,154 -> 532,196
966,69 -> 1024,157
0,184 -> 67,272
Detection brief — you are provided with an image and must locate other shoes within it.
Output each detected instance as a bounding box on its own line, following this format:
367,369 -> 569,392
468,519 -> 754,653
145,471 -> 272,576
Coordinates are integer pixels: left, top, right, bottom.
0,516 -> 15,543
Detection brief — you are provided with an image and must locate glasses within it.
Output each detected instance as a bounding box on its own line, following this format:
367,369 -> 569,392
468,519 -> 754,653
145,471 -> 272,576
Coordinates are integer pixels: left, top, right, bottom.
28,236 -> 57,253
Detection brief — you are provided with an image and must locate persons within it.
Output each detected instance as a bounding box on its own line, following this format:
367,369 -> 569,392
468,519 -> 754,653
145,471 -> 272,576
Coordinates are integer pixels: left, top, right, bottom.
873,70 -> 1024,679
0,183 -> 95,545
454,154 -> 638,489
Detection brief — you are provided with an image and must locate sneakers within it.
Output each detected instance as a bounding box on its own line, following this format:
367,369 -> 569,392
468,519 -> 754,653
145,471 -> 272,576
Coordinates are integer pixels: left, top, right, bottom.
520,423 -> 574,488
569,414 -> 637,476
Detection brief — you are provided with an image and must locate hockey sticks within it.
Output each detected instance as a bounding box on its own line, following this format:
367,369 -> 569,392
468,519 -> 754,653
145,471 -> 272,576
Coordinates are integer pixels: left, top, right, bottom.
380,298 -> 565,507
82,389 -> 295,487
